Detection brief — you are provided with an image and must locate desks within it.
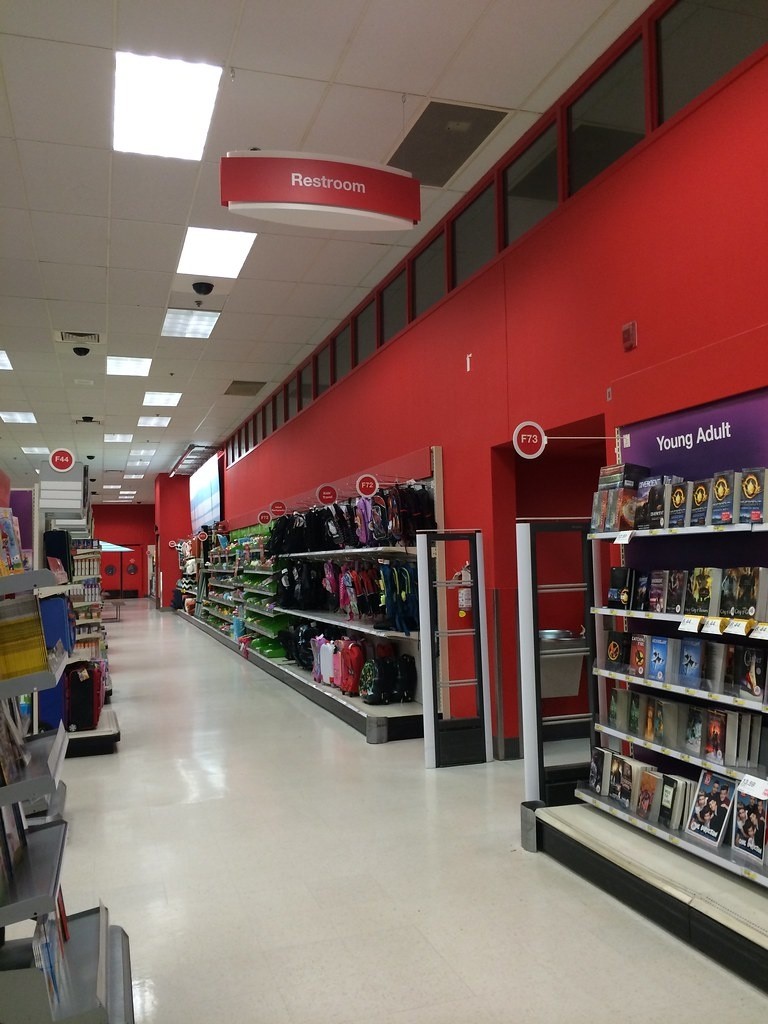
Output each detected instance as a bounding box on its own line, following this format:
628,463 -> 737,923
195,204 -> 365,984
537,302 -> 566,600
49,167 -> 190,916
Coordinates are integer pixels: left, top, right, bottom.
101,592 -> 110,596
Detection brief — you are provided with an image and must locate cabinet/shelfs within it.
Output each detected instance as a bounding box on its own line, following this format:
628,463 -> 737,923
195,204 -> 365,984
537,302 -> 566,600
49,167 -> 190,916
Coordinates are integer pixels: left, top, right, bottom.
73,547 -> 103,705
8,464 -> 122,760
175,444 -> 452,746
0,569 -> 137,1024
534,387 -> 768,995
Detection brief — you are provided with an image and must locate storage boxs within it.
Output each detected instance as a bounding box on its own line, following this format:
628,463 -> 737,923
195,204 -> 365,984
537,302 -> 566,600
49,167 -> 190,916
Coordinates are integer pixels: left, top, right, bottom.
65,557 -> 101,659
78,539 -> 84,555
598,480 -> 639,490
84,539 -> 93,555
598,473 -> 644,485
599,463 -> 650,477
72,538 -> 79,555
92,539 -> 100,555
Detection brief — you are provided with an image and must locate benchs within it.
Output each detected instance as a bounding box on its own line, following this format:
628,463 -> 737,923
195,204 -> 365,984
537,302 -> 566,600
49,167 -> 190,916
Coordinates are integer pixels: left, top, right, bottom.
111,601 -> 126,623
